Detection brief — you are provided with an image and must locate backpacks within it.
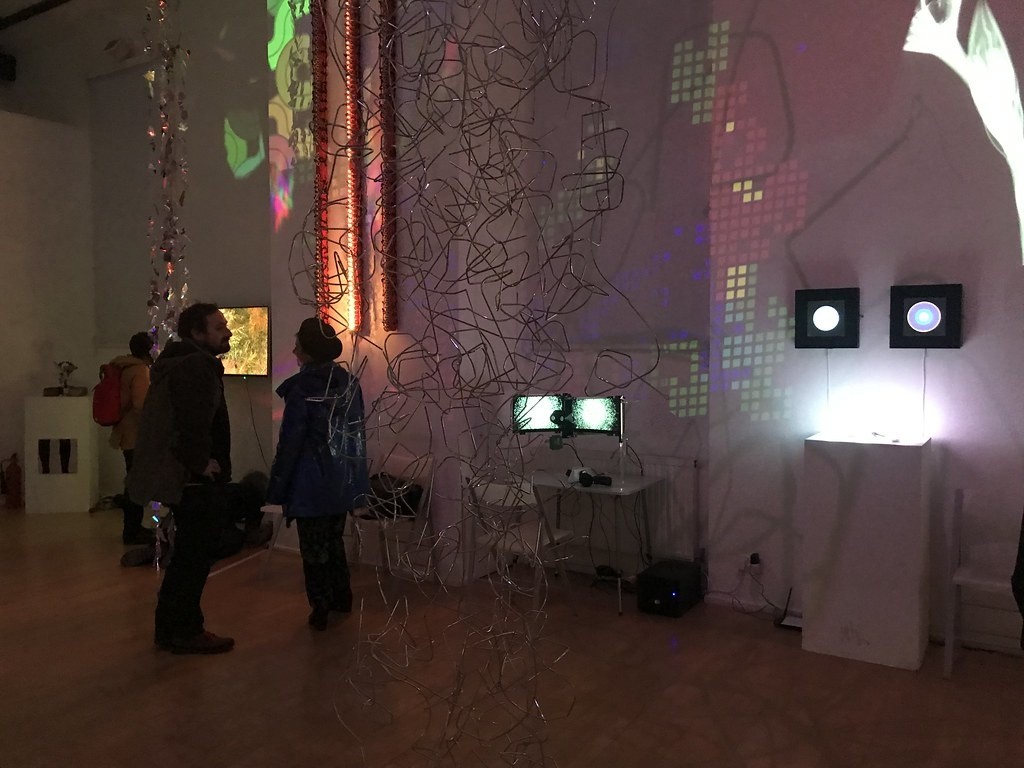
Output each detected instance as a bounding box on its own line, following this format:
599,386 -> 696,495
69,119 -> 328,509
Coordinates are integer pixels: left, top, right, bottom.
91,363 -> 137,426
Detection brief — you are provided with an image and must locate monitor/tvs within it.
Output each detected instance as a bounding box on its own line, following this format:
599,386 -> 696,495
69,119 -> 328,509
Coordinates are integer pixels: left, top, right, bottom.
564,394 -> 625,436
215,305 -> 271,377
510,392 -> 567,433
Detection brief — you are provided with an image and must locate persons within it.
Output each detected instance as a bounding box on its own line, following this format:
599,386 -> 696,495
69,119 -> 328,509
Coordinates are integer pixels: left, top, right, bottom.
146,302 -> 235,656
264,317 -> 370,633
120,470 -> 274,568
108,332 -> 158,546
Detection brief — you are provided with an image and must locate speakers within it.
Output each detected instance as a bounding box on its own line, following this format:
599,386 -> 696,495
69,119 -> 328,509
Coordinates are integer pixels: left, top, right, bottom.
634,559 -> 703,618
217,482 -> 249,560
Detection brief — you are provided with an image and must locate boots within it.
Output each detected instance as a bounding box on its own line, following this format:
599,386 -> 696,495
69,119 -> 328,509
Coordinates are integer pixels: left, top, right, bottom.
328,587 -> 352,612
308,597 -> 328,630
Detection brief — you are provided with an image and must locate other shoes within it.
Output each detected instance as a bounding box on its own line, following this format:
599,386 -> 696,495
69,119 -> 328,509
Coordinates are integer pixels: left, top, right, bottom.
123,525 -> 170,544
155,627 -> 234,654
120,546 -> 173,569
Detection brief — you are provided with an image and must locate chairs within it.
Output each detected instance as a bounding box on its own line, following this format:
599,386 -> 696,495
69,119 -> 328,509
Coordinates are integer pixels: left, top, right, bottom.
350,456 -> 436,576
464,475 -> 574,617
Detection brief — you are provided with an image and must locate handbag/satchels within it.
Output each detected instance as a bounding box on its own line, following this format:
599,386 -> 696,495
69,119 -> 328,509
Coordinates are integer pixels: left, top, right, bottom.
350,471 -> 423,520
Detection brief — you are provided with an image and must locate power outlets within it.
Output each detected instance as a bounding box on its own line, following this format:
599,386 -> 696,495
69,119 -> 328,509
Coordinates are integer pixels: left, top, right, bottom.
744,555 -> 764,576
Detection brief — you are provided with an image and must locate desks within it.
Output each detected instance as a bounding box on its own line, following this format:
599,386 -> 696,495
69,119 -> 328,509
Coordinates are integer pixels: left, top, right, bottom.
26,395 -> 99,514
529,465 -> 666,616
